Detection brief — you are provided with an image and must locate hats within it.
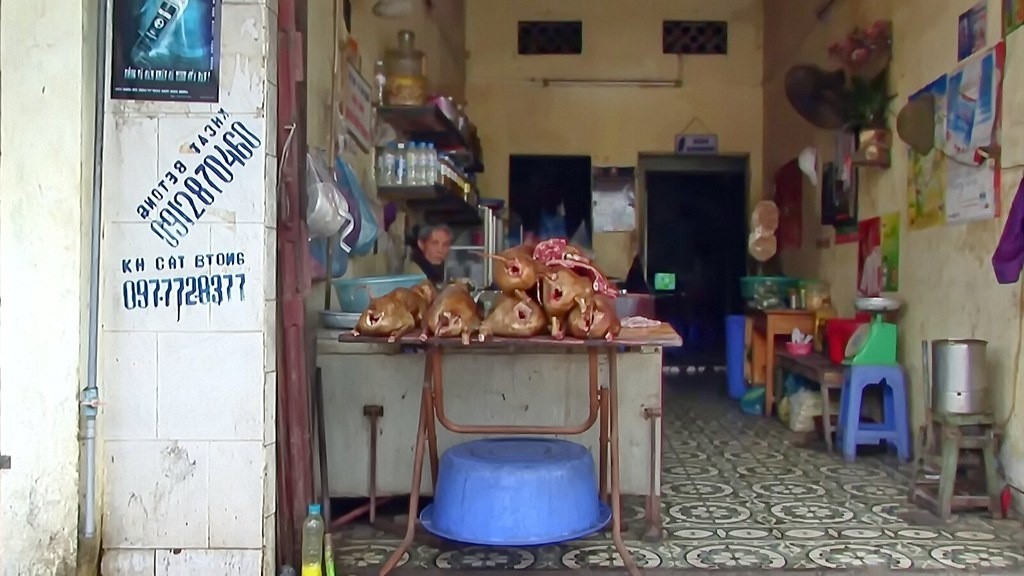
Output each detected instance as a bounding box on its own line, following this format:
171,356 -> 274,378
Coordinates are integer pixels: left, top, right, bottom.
897,93 -> 935,157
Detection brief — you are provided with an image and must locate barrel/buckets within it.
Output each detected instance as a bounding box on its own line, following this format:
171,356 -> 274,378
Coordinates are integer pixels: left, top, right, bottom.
931,337 -> 990,415
825,318 -> 865,365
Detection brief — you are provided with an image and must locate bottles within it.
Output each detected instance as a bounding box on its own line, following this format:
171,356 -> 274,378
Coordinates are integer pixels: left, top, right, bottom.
382,140 -> 474,205
787,288 -> 806,309
374,30 -> 427,107
813,298 -> 838,353
302,504 -> 325,576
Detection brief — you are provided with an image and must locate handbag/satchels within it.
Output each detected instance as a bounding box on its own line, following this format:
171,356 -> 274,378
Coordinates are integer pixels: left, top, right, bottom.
305,152 -> 379,285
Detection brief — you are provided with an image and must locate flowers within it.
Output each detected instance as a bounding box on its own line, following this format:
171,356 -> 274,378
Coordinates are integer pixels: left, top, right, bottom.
826,14 -> 892,126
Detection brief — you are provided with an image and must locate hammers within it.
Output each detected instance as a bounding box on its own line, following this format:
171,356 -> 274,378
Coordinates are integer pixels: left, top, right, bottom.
364,405 -> 383,523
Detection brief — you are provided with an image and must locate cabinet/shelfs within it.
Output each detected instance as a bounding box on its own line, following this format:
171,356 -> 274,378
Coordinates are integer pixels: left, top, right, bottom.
375,94 -> 485,230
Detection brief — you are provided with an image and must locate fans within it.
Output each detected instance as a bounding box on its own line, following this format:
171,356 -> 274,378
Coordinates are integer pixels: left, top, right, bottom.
785,63 -> 850,129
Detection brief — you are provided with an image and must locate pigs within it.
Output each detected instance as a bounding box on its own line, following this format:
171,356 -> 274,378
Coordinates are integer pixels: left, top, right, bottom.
470,242 -> 539,297
475,288 -> 546,342
350,281 -> 432,343
558,293 -> 623,341
419,280 -> 481,345
541,267 -> 592,338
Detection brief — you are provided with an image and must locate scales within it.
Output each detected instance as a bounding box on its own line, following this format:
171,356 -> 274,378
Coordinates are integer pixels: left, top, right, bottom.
841,297 -> 900,365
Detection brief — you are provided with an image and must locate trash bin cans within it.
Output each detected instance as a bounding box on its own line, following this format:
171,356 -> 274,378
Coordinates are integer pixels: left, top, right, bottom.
727,313 -> 751,400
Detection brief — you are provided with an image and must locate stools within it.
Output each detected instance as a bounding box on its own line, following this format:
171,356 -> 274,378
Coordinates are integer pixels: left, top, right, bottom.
837,362 -> 911,464
907,409 -> 1002,521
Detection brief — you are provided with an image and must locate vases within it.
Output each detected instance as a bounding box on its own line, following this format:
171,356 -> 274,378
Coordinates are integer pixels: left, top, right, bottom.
859,129 -> 895,170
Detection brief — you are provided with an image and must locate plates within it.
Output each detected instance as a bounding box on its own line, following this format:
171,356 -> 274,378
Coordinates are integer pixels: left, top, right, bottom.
317,311 -> 362,328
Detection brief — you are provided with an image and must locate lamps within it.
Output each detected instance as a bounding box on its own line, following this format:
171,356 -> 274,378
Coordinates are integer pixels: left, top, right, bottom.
543,78 -> 683,86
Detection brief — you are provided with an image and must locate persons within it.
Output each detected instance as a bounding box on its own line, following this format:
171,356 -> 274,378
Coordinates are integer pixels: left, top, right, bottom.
413,223 -> 451,287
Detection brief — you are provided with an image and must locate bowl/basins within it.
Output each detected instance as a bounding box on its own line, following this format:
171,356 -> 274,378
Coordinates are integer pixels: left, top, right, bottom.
785,342 -> 812,355
419,436 -> 613,548
328,274 -> 427,313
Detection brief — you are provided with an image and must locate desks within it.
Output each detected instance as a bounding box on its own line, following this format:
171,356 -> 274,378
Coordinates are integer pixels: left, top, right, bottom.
337,320 -> 685,576
747,308 -> 815,419
776,350 -> 889,454
